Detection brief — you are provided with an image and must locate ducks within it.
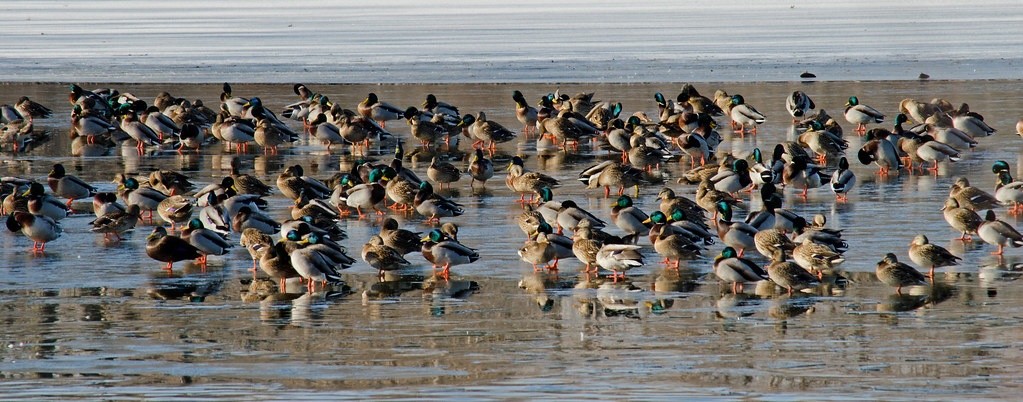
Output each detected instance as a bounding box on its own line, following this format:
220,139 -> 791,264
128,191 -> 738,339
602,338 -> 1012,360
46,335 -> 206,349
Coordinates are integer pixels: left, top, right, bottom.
0,81 -> 1023,295
